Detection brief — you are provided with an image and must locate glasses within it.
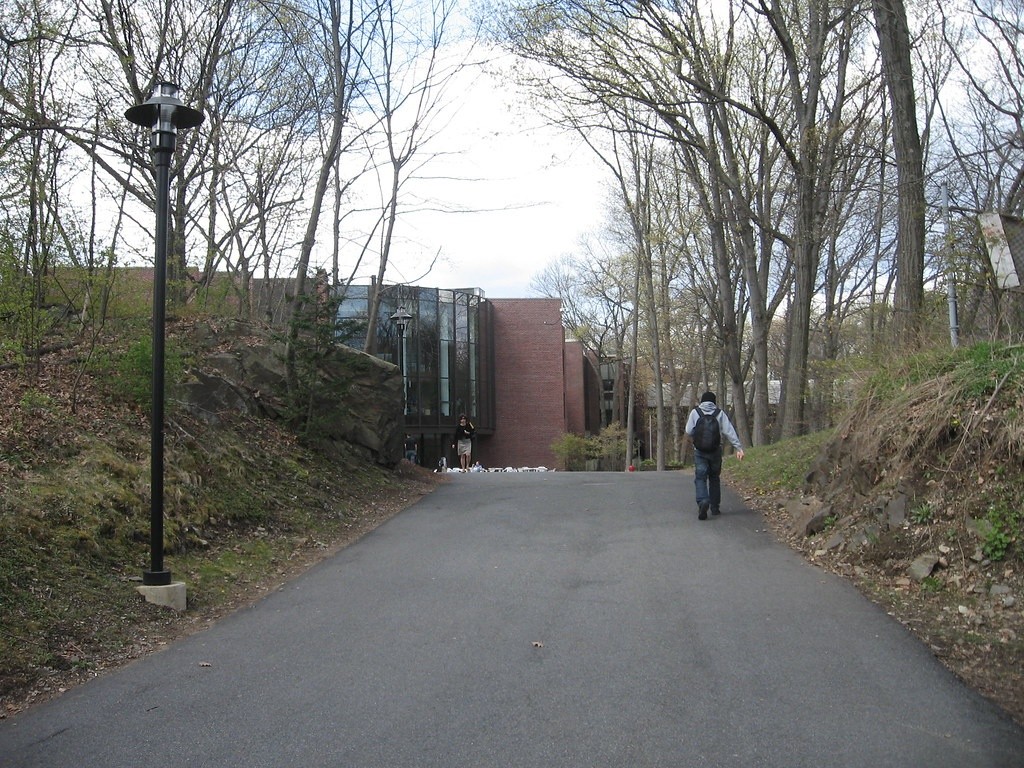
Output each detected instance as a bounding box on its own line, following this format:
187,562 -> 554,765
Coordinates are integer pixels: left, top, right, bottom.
460,418 -> 466,420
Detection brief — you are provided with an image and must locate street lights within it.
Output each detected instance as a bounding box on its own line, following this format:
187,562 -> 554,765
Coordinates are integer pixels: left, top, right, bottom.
122,79 -> 205,586
390,307 -> 412,459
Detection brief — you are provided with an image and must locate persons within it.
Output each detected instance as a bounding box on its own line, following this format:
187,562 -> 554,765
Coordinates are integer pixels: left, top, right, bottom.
452,413 -> 475,473
686,392 -> 745,520
405,433 -> 417,464
474,461 -> 483,472
440,454 -> 447,473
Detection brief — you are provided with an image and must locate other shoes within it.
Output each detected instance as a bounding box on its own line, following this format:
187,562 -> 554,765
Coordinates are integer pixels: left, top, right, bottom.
711,508 -> 721,515
461,468 -> 470,473
698,501 -> 709,520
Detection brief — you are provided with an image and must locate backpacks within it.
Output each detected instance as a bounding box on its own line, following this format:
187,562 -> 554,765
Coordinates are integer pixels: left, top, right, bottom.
692,407 -> 721,452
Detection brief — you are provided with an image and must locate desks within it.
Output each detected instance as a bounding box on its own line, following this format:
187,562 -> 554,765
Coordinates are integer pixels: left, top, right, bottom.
525,468 -> 536,473
487,468 -> 502,471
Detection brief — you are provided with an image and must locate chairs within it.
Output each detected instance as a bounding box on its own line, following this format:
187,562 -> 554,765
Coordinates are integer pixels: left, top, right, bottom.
435,465 -> 556,472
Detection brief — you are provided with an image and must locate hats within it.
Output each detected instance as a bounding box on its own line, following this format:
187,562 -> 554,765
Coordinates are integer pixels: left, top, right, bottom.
701,392 -> 717,405
